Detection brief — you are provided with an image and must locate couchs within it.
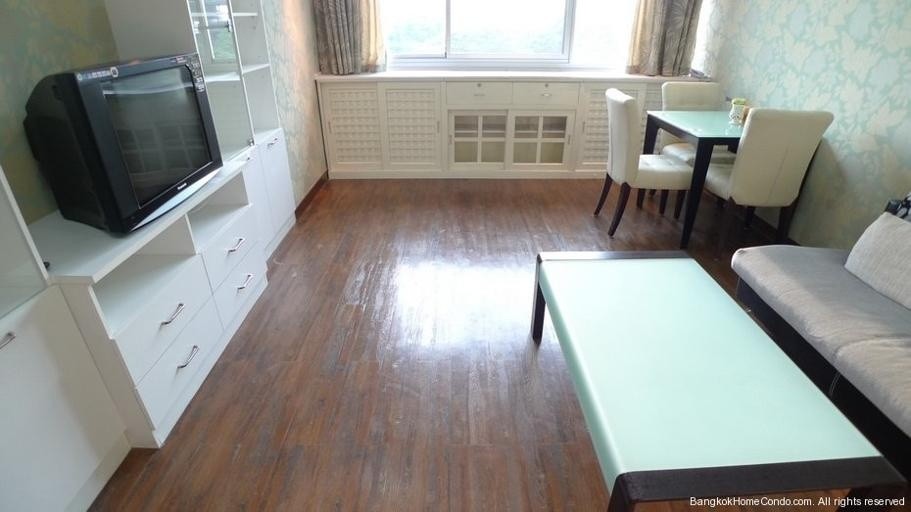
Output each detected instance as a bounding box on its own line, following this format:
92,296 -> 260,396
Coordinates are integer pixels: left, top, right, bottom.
731,193 -> 911,480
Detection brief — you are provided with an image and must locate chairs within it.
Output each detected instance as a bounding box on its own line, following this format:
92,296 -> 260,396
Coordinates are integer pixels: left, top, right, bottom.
704,107 -> 834,248
594,88 -> 695,237
650,82 -> 728,220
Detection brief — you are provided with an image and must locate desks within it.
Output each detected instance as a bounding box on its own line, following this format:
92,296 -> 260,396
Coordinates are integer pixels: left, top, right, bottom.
531,250 -> 910,512
636,111 -> 815,249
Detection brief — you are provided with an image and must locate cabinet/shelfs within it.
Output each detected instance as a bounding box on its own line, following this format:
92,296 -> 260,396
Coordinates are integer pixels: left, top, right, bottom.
315,80 -> 441,181
581,81 -> 664,180
0,166 -> 133,512
441,80 -> 581,181
29,160 -> 269,448
103,0 -> 297,259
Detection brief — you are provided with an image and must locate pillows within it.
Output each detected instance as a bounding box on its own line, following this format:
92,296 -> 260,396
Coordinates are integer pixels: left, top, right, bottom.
843,211 -> 911,312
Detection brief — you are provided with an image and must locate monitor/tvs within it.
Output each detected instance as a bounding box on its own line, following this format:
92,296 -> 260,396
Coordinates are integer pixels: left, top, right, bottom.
22,53 -> 224,235
